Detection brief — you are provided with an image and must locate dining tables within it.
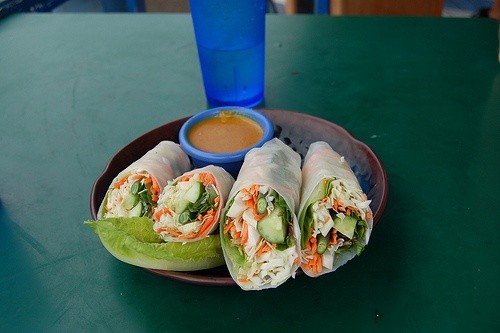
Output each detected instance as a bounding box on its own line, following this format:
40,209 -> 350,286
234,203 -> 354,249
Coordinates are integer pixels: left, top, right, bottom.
1,9 -> 499,333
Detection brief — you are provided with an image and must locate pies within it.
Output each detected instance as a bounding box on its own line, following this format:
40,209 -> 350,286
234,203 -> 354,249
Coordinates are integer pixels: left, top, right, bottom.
96,139 -> 190,221
299,142 -> 374,278
152,165 -> 236,244
219,138 -> 302,292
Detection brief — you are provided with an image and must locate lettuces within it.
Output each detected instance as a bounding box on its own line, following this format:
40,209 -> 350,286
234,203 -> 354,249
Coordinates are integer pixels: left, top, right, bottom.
82,216 -> 226,272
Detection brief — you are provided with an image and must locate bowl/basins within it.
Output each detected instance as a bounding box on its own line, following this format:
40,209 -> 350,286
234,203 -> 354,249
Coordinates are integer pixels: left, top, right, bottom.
179,107 -> 273,176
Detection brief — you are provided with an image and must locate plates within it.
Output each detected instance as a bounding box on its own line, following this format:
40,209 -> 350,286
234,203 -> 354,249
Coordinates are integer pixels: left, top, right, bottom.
90,105 -> 386,288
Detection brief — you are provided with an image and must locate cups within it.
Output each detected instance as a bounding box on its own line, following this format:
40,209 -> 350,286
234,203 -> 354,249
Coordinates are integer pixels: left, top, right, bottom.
190,0 -> 268,110
